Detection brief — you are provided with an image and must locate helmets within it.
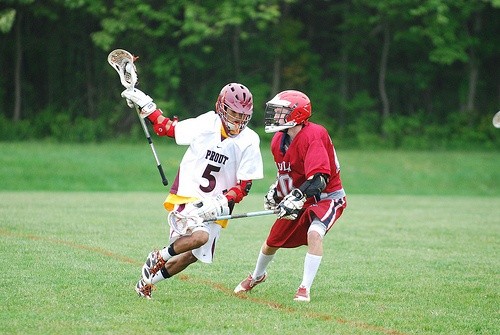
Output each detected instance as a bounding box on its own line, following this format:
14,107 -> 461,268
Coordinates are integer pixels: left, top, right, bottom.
216,83 -> 253,138
264,89 -> 312,133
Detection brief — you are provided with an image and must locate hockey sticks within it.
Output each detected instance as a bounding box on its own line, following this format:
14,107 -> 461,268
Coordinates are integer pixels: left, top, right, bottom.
107,47 -> 168,186
168,209 -> 287,238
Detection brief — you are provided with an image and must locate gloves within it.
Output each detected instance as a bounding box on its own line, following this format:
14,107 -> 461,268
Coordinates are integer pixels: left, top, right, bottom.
121,89 -> 155,119
193,195 -> 230,221
276,188 -> 308,220
263,181 -> 280,211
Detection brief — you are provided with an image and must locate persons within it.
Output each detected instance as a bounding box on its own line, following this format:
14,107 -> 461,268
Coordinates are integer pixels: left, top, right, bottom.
232,91 -> 348,303
121,83 -> 264,300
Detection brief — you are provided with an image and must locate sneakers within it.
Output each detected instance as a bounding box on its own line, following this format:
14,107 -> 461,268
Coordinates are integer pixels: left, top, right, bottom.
294,287 -> 310,301
135,279 -> 152,299
142,250 -> 166,286
234,272 -> 266,296
